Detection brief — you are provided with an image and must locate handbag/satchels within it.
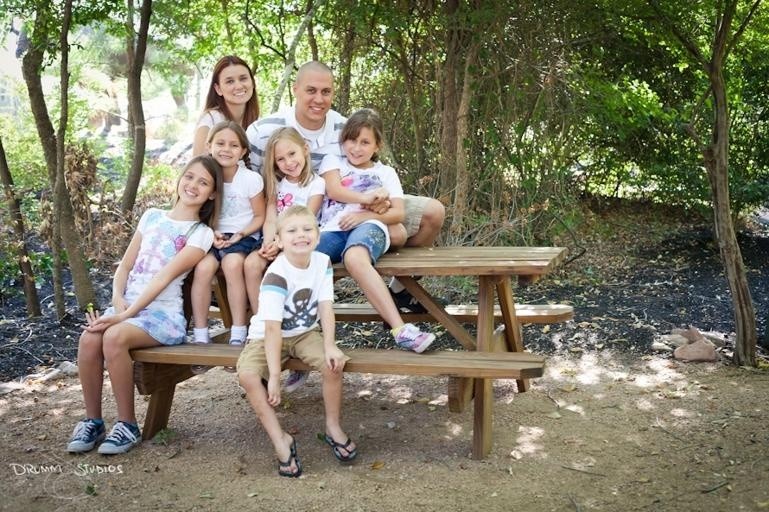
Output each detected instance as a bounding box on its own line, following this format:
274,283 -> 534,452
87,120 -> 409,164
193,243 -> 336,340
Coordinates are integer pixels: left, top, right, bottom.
158,131 -> 194,167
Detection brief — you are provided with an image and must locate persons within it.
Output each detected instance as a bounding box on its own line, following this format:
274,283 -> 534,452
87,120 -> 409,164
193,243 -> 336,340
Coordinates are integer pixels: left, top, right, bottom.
66,154 -> 224,458
235,204 -> 360,479
243,124 -> 327,316
191,54 -> 258,161
282,107 -> 435,393
225,59 -> 447,314
183,119 -> 267,347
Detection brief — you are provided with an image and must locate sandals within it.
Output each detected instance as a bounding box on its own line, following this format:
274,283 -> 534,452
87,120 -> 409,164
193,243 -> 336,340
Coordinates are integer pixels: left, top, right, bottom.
277,431 -> 302,478
324,433 -> 358,461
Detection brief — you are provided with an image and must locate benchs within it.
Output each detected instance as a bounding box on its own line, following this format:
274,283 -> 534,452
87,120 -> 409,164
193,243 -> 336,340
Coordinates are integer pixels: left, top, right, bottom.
131,342 -> 548,459
210,302 -> 578,395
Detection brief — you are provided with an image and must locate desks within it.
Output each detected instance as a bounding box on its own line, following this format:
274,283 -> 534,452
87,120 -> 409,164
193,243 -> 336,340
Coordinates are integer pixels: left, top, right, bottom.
140,243 -> 573,458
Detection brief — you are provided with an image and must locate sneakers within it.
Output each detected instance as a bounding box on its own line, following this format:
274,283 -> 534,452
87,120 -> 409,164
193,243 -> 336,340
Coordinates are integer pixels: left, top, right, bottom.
224,338 -> 246,372
390,323 -> 436,354
97,420 -> 142,454
284,371 -> 305,394
67,418 -> 105,452
190,338 -> 213,375
387,284 -> 448,313
